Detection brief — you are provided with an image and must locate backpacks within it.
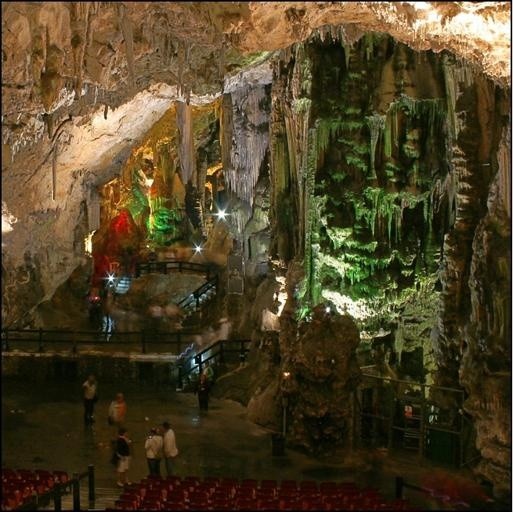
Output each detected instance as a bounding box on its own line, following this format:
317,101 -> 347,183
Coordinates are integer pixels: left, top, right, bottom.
150,438 -> 163,460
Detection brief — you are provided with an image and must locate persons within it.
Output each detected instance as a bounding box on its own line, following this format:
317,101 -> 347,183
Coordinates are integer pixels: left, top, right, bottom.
108,391 -> 127,444
143,425 -> 162,480
143,423 -> 156,439
114,428 -> 131,488
157,421 -> 178,478
81,374 -> 96,425
202,361 -> 215,400
192,375 -> 211,417
